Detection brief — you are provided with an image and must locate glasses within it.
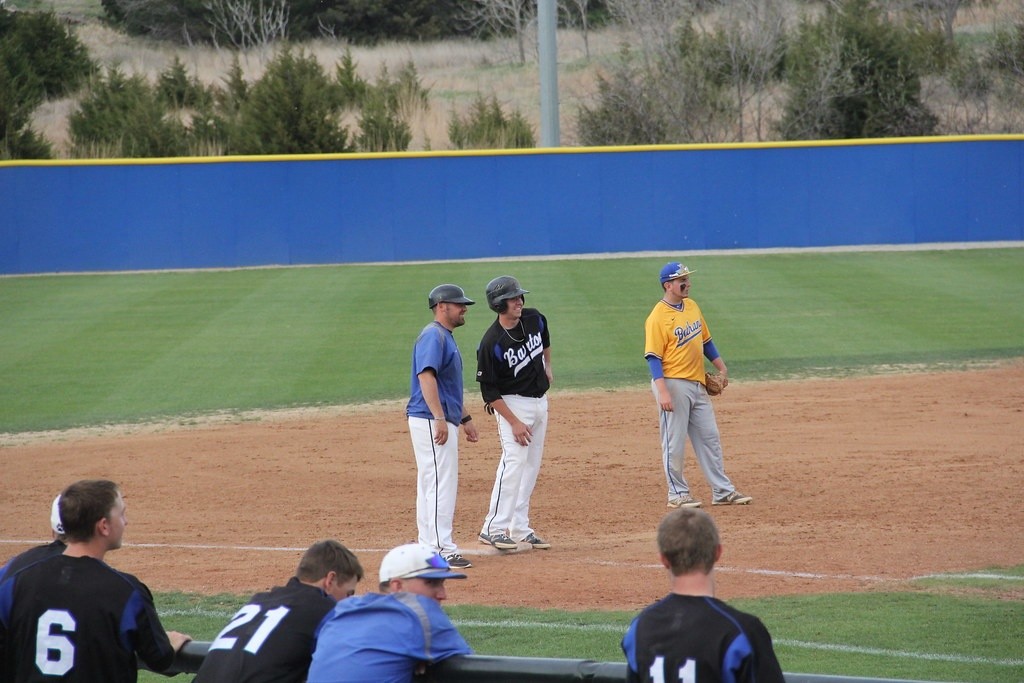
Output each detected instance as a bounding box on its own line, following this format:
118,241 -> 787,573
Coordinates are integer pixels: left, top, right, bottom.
390,555 -> 450,580
660,267 -> 688,279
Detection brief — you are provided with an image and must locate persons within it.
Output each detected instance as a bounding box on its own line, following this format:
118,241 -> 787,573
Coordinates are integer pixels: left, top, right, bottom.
190,541 -> 363,683
476,276 -> 553,549
644,262 -> 754,508
307,543 -> 474,683
406,284 -> 479,569
0,479 -> 193,683
620,507 -> 784,683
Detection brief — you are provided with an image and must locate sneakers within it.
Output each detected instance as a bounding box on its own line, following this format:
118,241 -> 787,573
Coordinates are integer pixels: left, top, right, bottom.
712,491 -> 753,506
478,533 -> 517,549
442,554 -> 473,568
519,532 -> 551,549
666,496 -> 702,508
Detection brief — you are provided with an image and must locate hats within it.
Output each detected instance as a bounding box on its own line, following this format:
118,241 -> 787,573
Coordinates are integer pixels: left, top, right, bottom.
659,262 -> 696,283
379,543 -> 467,584
50,494 -> 65,534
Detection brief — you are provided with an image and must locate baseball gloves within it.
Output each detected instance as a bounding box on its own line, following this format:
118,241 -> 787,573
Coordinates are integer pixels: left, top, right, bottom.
704,373 -> 728,396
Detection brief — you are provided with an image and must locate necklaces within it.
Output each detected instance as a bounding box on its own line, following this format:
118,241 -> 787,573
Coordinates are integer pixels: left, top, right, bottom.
498,318 -> 525,342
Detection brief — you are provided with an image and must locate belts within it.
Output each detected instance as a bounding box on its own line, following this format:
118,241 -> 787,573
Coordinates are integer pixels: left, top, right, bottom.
514,393 -> 541,398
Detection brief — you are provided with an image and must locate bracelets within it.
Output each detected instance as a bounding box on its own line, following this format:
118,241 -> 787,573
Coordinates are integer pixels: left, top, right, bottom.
460,415 -> 471,424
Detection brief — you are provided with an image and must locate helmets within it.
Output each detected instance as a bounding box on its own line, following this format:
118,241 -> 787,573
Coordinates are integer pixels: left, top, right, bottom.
428,284 -> 475,309
485,276 -> 530,313
